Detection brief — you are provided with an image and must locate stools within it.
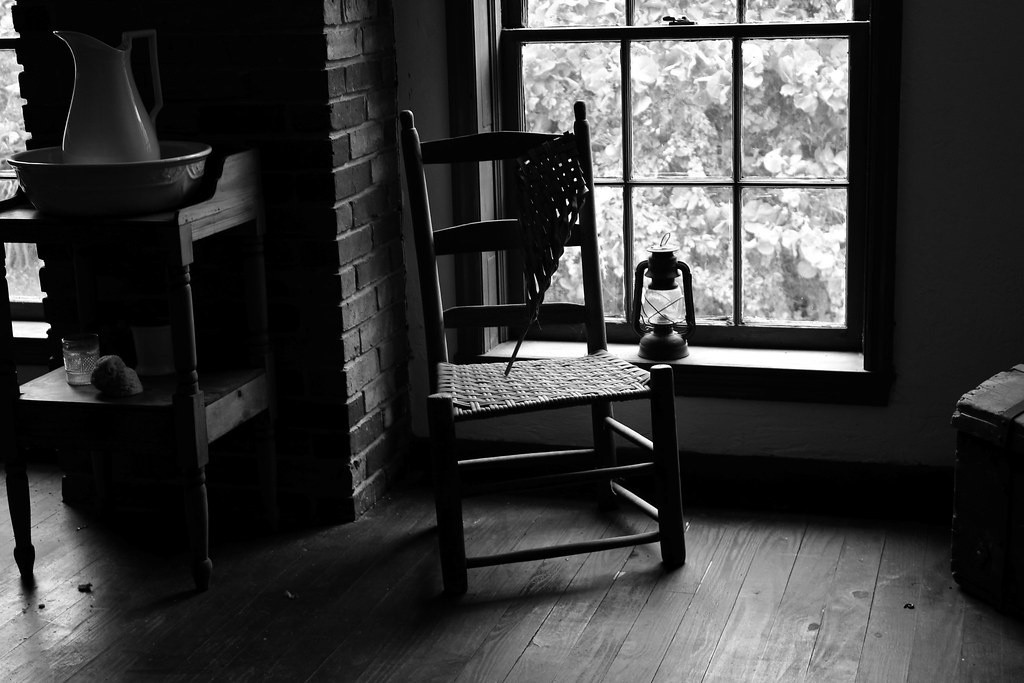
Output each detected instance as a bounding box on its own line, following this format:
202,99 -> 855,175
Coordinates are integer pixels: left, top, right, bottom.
948,363 -> 1024,624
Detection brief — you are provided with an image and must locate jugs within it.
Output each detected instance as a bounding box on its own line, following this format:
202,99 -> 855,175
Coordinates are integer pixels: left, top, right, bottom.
52,27 -> 163,166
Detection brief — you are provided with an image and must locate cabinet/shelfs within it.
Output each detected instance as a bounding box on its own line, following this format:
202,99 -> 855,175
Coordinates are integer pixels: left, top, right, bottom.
1,143 -> 285,593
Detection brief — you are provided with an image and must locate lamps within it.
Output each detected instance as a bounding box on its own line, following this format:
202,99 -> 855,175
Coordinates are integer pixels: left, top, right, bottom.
631,231 -> 697,363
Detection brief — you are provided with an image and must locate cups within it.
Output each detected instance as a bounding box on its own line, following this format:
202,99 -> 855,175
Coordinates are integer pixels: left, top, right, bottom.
61,333 -> 100,387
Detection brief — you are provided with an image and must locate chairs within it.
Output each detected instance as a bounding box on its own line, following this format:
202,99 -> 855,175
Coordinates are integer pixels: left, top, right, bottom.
400,101 -> 697,601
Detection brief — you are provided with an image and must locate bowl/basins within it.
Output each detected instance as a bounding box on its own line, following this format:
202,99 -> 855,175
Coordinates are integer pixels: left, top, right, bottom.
7,142 -> 212,219
